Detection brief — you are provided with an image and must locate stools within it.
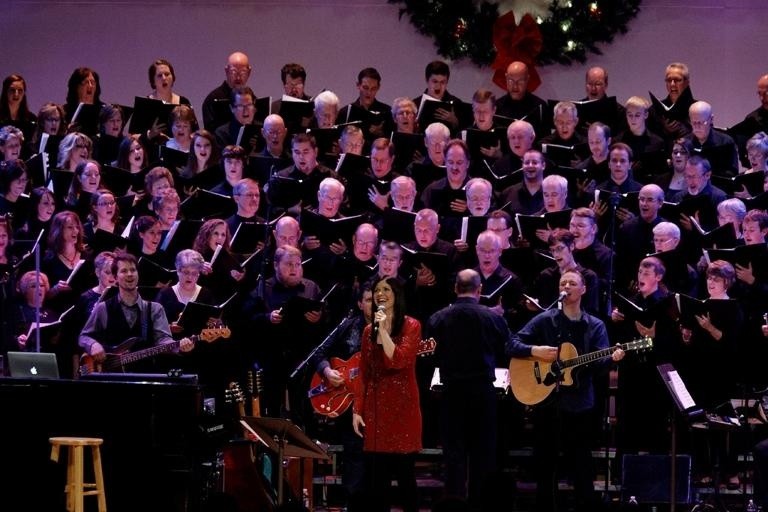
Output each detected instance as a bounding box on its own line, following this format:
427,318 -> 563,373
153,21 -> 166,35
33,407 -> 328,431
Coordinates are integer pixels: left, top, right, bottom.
49,436 -> 107,512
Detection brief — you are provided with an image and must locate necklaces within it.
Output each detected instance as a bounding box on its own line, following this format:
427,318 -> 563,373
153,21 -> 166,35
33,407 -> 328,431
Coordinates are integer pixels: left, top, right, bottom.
673,172 -> 684,185
157,92 -> 171,104
58,247 -> 77,270
175,286 -> 198,303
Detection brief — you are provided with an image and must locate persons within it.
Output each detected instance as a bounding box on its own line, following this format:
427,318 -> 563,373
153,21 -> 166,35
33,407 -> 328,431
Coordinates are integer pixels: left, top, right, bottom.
213,86 -> 265,155
131,216 -> 173,290
154,189 -> 181,248
406,122 -> 451,195
78,188 -> 124,256
337,126 -> 364,156
451,177 -> 493,252
538,102 -> 588,166
647,63 -> 696,136
272,216 -> 301,247
493,121 -> 536,192
350,276 -> 421,511
97,103 -> 127,162
163,105 -> 196,178
678,260 -> 741,490
11,271 -> 64,352
651,222 -> 698,299
685,101 -> 738,176
348,221 -> 379,283
617,184 -> 667,238
421,269 -> 558,511
263,133 -> 346,216
655,137 -> 693,202
73,251 -> 116,376
193,218 -> 247,283
122,59 -> 200,146
210,144 -> 247,197
113,136 -> 148,207
271,62 -> 313,127
78,252 -> 195,373
26,103 -> 65,155
314,281 -> 375,388
313,91 -> 343,153
611,256 -> 675,484
0,126 -> 24,161
0,212 -> 19,285
717,198 -> 745,246
334,67 -> 394,143
16,186 -> 55,243
572,121 -> 612,201
569,208 -> 615,271
60,67 -> 102,135
156,248 -> 225,337
614,97 -> 665,172
56,132 -> 91,171
462,89 -> 508,170
64,157 -> 105,206
499,149 -> 545,215
588,143 -> 643,222
517,175 -> 574,247
202,51 -> 252,134
365,242 -> 405,292
257,112 -> 291,158
0,74 -> 37,140
179,131 -> 216,197
524,229 -> 598,314
420,139 -> 474,215
412,60 -> 466,138
745,73 -> 768,132
0,158 -> 29,235
733,131 -> 768,210
669,156 -> 726,230
246,245 -> 331,416
225,178 -> 270,252
581,70 -> 626,128
491,61 -> 550,134
362,138 -> 400,214
506,269 -> 626,511
733,209 -> 768,286
302,178 -> 354,262
404,209 -> 456,295
390,96 -> 424,164
474,229 -> 519,316
485,211 -> 515,250
133,166 -> 174,219
376,176 -> 418,232
40,211 -> 93,300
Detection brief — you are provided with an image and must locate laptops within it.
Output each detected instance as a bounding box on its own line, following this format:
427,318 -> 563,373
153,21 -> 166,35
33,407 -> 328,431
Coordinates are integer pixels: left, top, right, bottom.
7,351 -> 59,378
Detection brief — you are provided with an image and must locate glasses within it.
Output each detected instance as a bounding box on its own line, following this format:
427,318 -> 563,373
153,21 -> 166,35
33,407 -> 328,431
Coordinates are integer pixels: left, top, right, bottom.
96,200 -> 116,207
231,103 -> 255,111
74,144 -> 90,149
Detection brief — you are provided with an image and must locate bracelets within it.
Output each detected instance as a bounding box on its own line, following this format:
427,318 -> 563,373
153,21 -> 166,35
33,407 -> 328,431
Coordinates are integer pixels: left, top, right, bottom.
147,129 -> 151,140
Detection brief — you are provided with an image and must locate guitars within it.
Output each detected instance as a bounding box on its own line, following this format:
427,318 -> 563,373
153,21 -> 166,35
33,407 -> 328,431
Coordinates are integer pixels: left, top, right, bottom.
306,337 -> 438,421
77,324 -> 233,379
507,333 -> 654,409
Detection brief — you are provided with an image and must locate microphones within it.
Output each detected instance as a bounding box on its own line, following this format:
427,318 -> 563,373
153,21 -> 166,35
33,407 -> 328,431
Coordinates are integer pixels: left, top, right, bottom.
373,305 -> 386,332
547,291 -> 567,311
